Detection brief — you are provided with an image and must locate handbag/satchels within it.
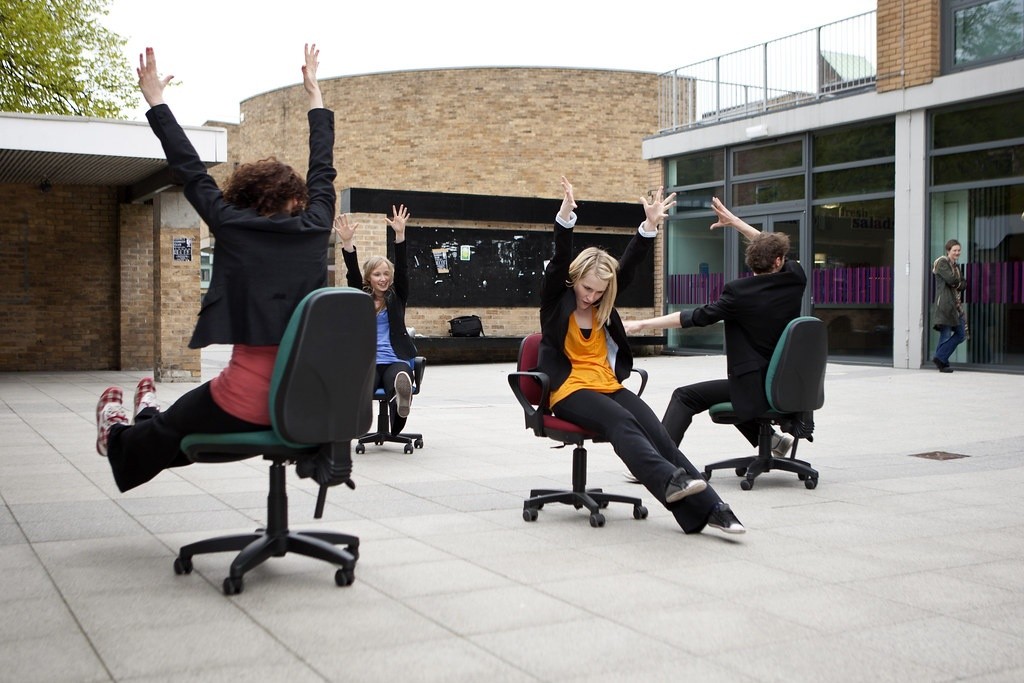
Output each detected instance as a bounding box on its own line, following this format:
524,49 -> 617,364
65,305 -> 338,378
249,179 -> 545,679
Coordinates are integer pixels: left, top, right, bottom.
447,315 -> 485,338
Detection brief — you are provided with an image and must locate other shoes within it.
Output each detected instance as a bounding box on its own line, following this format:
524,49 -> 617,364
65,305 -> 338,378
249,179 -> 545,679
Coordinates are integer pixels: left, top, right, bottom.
933,358 -> 953,373
391,371 -> 412,418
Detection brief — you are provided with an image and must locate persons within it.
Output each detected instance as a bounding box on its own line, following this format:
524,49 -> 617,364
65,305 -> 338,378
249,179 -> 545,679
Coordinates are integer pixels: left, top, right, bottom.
622,197 -> 807,480
97,44 -> 337,493
930,240 -> 968,372
538,176 -> 746,535
332,204 -> 417,417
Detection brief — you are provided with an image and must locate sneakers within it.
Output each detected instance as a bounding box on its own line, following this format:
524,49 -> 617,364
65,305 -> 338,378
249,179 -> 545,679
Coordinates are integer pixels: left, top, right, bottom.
624,469 -> 642,484
708,502 -> 745,533
131,377 -> 160,426
665,467 -> 707,503
96,386 -> 129,456
771,436 -> 793,458
